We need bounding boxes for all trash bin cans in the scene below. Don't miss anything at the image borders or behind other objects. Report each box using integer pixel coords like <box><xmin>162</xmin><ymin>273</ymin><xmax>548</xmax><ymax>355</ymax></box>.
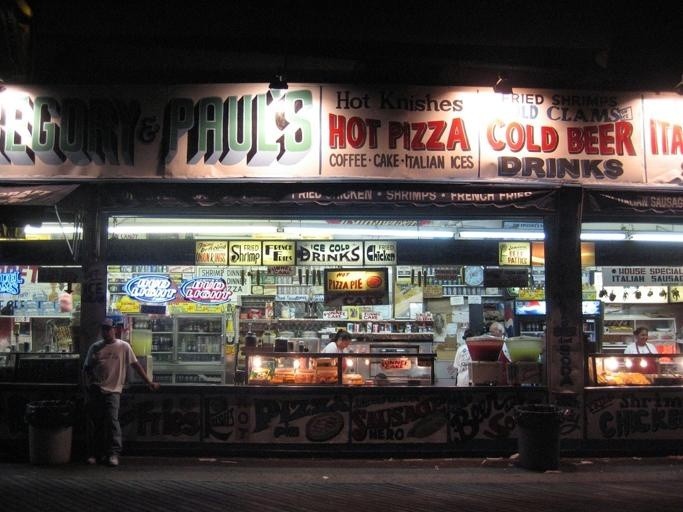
<box><xmin>27</xmin><ymin>400</ymin><xmax>73</xmax><ymax>465</ymax></box>
<box><xmin>513</xmin><ymin>404</ymin><xmax>564</xmax><ymax>472</ymax></box>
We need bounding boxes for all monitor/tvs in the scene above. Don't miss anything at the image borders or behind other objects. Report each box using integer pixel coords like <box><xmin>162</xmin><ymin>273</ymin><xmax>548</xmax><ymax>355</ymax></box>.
<box><xmin>323</xmin><ymin>267</ymin><xmax>390</xmax><ymax>306</ymax></box>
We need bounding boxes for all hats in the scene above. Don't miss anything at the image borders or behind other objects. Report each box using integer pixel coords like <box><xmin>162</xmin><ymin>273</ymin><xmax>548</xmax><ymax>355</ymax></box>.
<box><xmin>101</xmin><ymin>318</ymin><xmax>116</xmax><ymax>328</ymax></box>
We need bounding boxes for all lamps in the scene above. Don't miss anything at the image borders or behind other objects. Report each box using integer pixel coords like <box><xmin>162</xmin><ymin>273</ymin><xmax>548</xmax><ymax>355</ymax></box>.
<box><xmin>493</xmin><ymin>78</ymin><xmax>512</xmax><ymax>97</ymax></box>
<box><xmin>269</xmin><ymin>75</ymin><xmax>289</xmax><ymax>101</ymax></box>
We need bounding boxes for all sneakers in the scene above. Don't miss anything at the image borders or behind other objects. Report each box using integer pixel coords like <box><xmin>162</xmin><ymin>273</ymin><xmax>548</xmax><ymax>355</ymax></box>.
<box><xmin>107</xmin><ymin>455</ymin><xmax>118</xmax><ymax>466</ymax></box>
<box><xmin>86</xmin><ymin>457</ymin><xmax>96</xmax><ymax>465</ymax></box>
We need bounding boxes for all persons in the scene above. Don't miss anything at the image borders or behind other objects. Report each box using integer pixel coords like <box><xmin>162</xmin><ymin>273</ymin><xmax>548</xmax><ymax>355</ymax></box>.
<box><xmin>453</xmin><ymin>328</ymin><xmax>477</xmax><ymax>387</ymax></box>
<box><xmin>80</xmin><ymin>317</ymin><xmax>162</xmax><ymax>468</ymax></box>
<box><xmin>623</xmin><ymin>325</ymin><xmax>661</xmax><ymax>376</ymax></box>
<box><xmin>488</xmin><ymin>321</ymin><xmax>513</xmax><ymax>387</ymax></box>
<box><xmin>317</xmin><ymin>328</ymin><xmax>353</xmax><ymax>374</ymax></box>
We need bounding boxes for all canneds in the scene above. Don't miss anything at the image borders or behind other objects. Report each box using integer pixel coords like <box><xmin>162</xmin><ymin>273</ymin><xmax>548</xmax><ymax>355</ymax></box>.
<box><xmin>429</xmin><ymin>278</ymin><xmax>458</xmax><ymax>286</ymax></box>
<box><xmin>344</xmin><ymin>323</ymin><xmax>433</xmax><ymax>333</ymax></box>
<box><xmin>443</xmin><ymin>287</ymin><xmax>481</xmax><ymax>295</ymax></box>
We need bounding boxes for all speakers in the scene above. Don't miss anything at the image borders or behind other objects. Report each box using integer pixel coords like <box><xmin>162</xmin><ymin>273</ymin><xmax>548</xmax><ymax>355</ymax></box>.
<box><xmin>38</xmin><ymin>267</ymin><xmax>83</xmax><ymax>282</ymax></box>
<box><xmin>483</xmin><ymin>269</ymin><xmax>528</xmax><ymax>287</ymax></box>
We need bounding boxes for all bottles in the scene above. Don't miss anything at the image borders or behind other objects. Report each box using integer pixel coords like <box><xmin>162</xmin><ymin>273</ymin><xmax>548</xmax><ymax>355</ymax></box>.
<box><xmin>422</xmin><ymin>267</ymin><xmax>481</xmax><ymax>295</ymax></box>
<box><xmin>133</xmin><ymin>316</ymin><xmax>221</xmax><ymax>384</ymax></box>
<box><xmin>520</xmin><ymin>318</ymin><xmax>545</xmax><ymax>332</ymax></box>
<box><xmin>518</xmin><ymin>286</ymin><xmax>544</xmax><ymax>299</ymax></box>
<box><xmin>581</xmin><ymin>283</ymin><xmax>597</xmax><ymax>301</ymax></box>
